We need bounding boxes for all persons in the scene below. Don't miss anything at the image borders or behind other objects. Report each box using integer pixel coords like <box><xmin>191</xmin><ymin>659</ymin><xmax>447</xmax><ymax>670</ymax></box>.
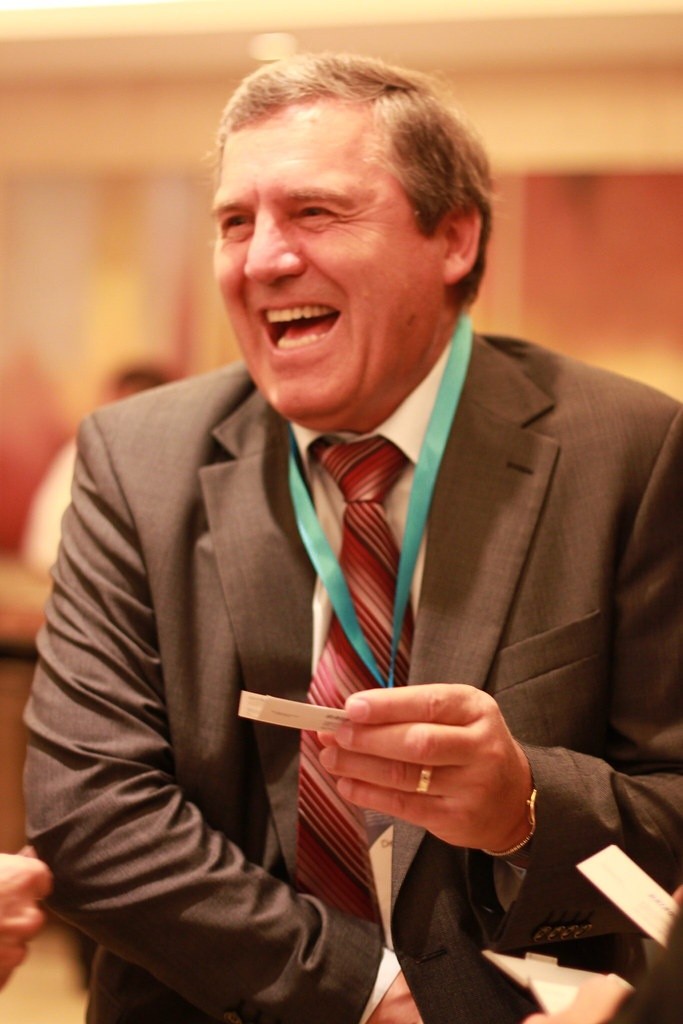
<box><xmin>24</xmin><ymin>367</ymin><xmax>165</xmax><ymax>583</ymax></box>
<box><xmin>23</xmin><ymin>56</ymin><xmax>683</xmax><ymax>1024</ymax></box>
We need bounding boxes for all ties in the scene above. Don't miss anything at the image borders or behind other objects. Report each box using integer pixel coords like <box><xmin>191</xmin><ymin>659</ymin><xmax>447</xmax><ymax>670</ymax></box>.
<box><xmin>295</xmin><ymin>432</ymin><xmax>413</xmax><ymax>949</ymax></box>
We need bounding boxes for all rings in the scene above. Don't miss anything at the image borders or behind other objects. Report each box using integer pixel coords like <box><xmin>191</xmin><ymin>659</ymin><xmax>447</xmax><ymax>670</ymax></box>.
<box><xmin>416</xmin><ymin>766</ymin><xmax>432</xmax><ymax>793</ymax></box>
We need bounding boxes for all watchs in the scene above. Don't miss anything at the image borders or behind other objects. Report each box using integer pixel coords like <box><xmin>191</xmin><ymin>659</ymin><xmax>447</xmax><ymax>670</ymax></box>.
<box><xmin>482</xmin><ymin>789</ymin><xmax>537</xmax><ymax>856</ymax></box>
